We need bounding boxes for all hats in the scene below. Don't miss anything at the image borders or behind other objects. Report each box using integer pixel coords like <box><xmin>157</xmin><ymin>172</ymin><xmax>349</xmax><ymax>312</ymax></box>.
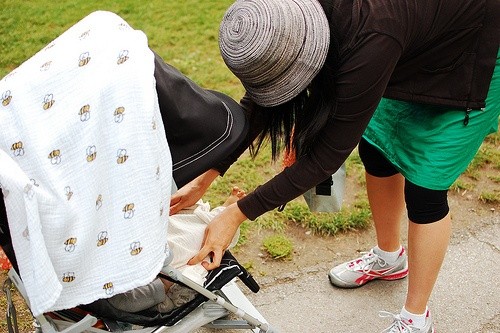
<box><xmin>217</xmin><ymin>0</ymin><xmax>331</xmax><ymax>108</ymax></box>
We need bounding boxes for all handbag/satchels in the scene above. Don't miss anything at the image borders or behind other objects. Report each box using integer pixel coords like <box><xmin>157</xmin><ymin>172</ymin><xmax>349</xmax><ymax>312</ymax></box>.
<box><xmin>302</xmin><ymin>162</ymin><xmax>347</xmax><ymax>214</ymax></box>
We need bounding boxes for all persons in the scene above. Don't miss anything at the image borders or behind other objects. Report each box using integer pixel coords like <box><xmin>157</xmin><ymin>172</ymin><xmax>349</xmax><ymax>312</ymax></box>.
<box><xmin>105</xmin><ymin>186</ymin><xmax>247</xmax><ymax>313</ymax></box>
<box><xmin>167</xmin><ymin>0</ymin><xmax>500</xmax><ymax>333</ymax></box>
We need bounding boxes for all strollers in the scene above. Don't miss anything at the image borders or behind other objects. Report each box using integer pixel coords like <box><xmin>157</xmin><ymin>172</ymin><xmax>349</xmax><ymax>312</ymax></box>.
<box><xmin>1</xmin><ymin>11</ymin><xmax>273</xmax><ymax>333</ymax></box>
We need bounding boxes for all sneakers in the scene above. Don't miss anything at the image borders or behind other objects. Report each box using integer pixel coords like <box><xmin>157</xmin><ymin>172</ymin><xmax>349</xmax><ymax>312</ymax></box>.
<box><xmin>327</xmin><ymin>245</ymin><xmax>408</xmax><ymax>289</ymax></box>
<box><xmin>385</xmin><ymin>309</ymin><xmax>436</xmax><ymax>333</ymax></box>
